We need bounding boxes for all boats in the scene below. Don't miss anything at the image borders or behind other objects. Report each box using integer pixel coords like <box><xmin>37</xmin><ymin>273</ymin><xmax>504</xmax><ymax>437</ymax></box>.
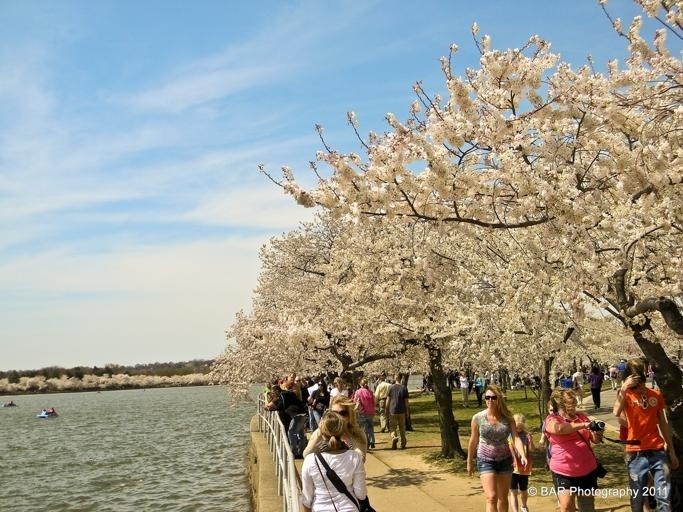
<box><xmin>37</xmin><ymin>407</ymin><xmax>58</xmax><ymax>419</ymax></box>
<box><xmin>1</xmin><ymin>401</ymin><xmax>17</xmax><ymax>408</ymax></box>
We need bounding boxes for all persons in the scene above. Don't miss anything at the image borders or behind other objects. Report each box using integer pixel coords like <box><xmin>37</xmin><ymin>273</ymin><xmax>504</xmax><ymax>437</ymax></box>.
<box><xmin>420</xmin><ymin>359</ymin><xmax>679</xmax><ymax>512</ymax></box>
<box><xmin>41</xmin><ymin>407</ymin><xmax>54</xmax><ymax>415</ymax></box>
<box><xmin>261</xmin><ymin>370</ymin><xmax>411</xmax><ymax>512</ymax></box>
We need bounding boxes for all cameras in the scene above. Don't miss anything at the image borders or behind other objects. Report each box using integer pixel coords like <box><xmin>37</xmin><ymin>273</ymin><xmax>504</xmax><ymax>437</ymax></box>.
<box><xmin>589</xmin><ymin>421</ymin><xmax>605</xmax><ymax>431</ymax></box>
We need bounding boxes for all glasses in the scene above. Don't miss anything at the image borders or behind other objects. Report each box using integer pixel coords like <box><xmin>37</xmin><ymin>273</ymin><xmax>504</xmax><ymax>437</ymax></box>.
<box><xmin>485</xmin><ymin>395</ymin><xmax>497</xmax><ymax>400</ymax></box>
<box><xmin>641</xmin><ymin>394</ymin><xmax>648</xmax><ymax>410</ymax></box>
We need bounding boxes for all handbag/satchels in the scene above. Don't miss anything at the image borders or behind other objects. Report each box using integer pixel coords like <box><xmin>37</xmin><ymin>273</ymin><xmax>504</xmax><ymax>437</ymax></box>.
<box><xmin>597</xmin><ymin>464</ymin><xmax>607</xmax><ymax>478</ymax></box>
